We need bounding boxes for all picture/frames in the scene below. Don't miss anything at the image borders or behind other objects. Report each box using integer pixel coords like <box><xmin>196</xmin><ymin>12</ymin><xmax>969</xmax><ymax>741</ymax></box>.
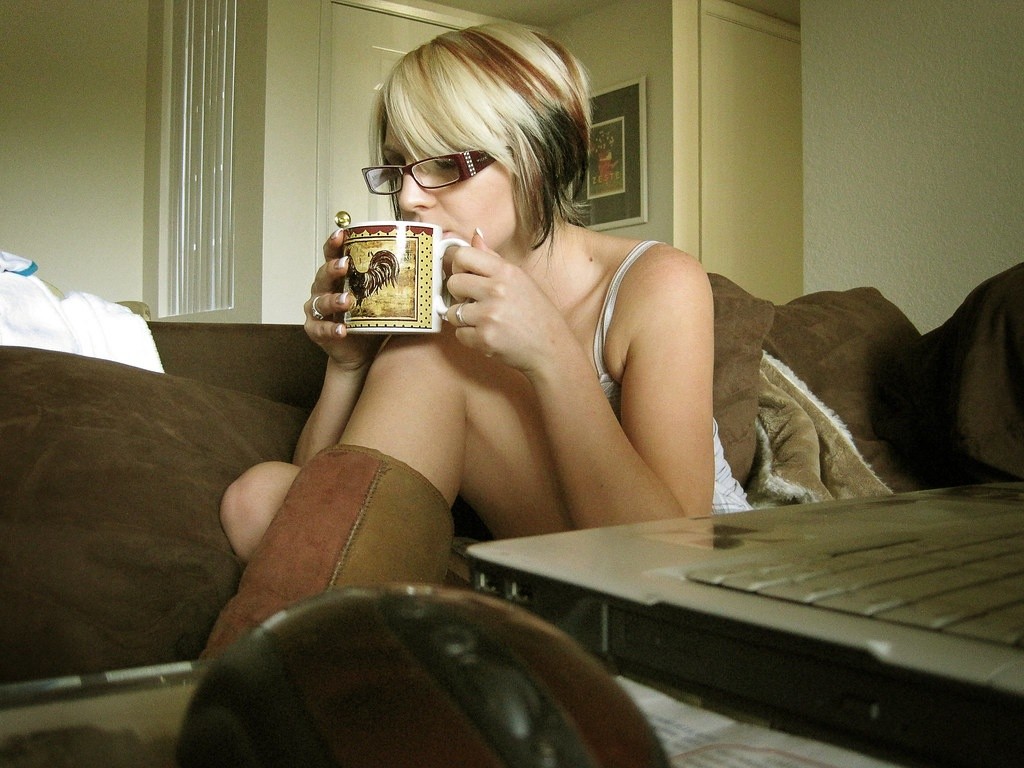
<box><xmin>569</xmin><ymin>76</ymin><xmax>649</xmax><ymax>232</ymax></box>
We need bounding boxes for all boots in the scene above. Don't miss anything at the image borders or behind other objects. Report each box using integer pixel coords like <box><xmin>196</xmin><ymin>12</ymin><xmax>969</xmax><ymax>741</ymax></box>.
<box><xmin>199</xmin><ymin>441</ymin><xmax>457</xmax><ymax>673</ymax></box>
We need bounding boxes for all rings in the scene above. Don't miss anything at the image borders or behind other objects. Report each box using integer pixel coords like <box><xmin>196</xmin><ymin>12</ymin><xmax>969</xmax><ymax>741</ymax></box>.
<box><xmin>455</xmin><ymin>302</ymin><xmax>468</xmax><ymax>327</ymax></box>
<box><xmin>312</xmin><ymin>296</ymin><xmax>326</xmax><ymax>321</ymax></box>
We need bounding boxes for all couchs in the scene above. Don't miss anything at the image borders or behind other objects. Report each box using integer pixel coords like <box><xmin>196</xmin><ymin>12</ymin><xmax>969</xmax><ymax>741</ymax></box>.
<box><xmin>0</xmin><ymin>261</ymin><xmax>1023</xmax><ymax>686</ymax></box>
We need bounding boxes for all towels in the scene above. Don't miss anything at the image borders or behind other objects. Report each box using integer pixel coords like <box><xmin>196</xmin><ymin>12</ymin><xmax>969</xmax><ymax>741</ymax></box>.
<box><xmin>2</xmin><ymin>249</ymin><xmax>165</xmax><ymax>371</ymax></box>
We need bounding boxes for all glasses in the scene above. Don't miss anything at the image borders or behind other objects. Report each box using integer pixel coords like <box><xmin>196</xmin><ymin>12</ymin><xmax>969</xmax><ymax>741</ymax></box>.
<box><xmin>362</xmin><ymin>151</ymin><xmax>496</xmax><ymax>195</ymax></box>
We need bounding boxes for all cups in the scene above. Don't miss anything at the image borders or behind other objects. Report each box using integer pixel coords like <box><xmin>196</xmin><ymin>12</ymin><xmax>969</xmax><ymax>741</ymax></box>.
<box><xmin>341</xmin><ymin>220</ymin><xmax>472</xmax><ymax>335</ymax></box>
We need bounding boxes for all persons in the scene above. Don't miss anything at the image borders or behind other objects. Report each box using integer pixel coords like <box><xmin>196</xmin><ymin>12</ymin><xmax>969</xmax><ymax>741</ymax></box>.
<box><xmin>201</xmin><ymin>20</ymin><xmax>752</xmax><ymax>668</ymax></box>
